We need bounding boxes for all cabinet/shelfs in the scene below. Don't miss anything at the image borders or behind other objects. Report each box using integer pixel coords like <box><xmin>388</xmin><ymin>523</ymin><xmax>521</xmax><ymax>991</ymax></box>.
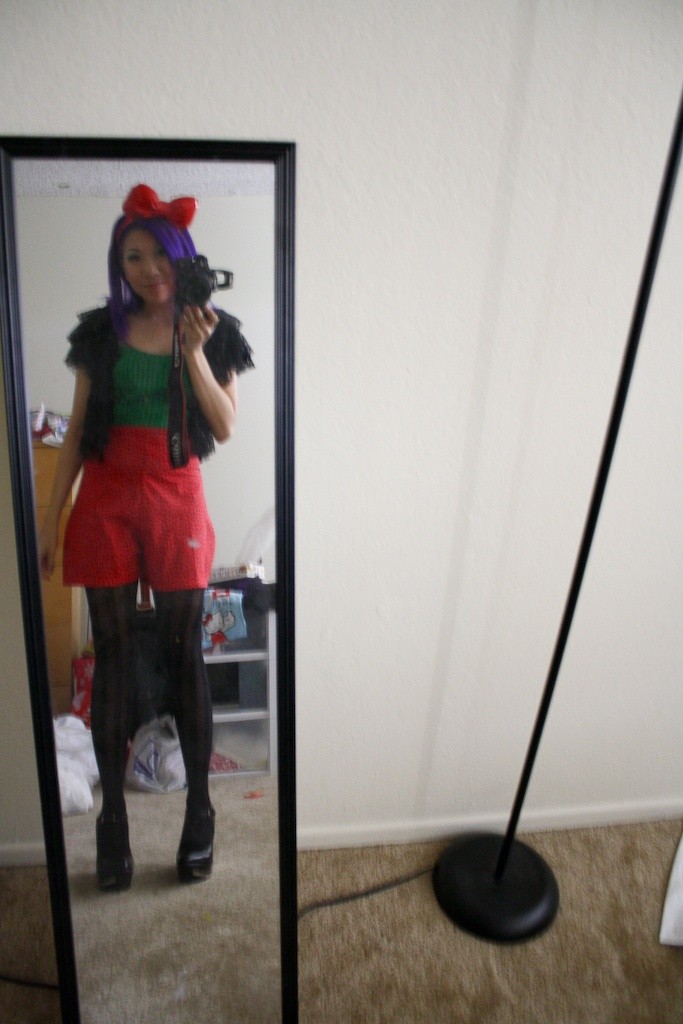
<box><xmin>204</xmin><ymin>610</ymin><xmax>278</xmax><ymax>777</ymax></box>
<box><xmin>33</xmin><ymin>440</ymin><xmax>87</xmax><ymax>718</ymax></box>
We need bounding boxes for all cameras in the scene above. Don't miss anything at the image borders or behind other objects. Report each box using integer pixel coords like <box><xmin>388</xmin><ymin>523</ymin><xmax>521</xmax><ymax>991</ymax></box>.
<box><xmin>176</xmin><ymin>255</ymin><xmax>234</xmax><ymax>307</ymax></box>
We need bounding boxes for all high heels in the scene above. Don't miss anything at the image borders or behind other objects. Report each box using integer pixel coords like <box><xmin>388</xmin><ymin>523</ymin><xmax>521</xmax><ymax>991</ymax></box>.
<box><xmin>174</xmin><ymin>809</ymin><xmax>216</xmax><ymax>886</ymax></box>
<box><xmin>93</xmin><ymin>813</ymin><xmax>134</xmax><ymax>892</ymax></box>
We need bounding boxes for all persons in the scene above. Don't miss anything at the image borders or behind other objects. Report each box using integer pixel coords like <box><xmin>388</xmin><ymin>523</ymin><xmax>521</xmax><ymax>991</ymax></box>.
<box><xmin>35</xmin><ymin>186</ymin><xmax>258</xmax><ymax>887</ymax></box>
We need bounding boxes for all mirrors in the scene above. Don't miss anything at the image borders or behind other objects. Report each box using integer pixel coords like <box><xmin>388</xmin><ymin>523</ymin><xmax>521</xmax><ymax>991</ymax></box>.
<box><xmin>0</xmin><ymin>133</ymin><xmax>300</xmax><ymax>1024</ymax></box>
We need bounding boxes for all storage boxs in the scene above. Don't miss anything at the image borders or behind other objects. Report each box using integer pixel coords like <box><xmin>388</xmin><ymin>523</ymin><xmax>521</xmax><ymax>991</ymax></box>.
<box><xmin>210</xmin><ymin>563</ymin><xmax>265</xmax><ymax>583</ymax></box>
